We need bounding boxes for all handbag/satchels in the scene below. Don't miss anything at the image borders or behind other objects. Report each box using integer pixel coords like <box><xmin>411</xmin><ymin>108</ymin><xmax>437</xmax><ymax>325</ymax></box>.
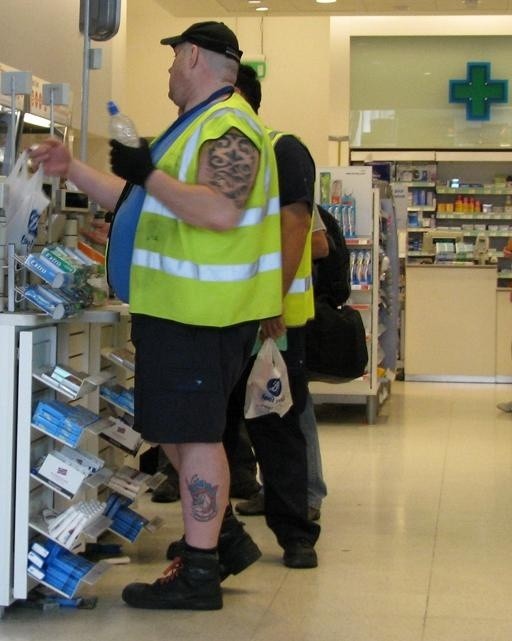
<box><xmin>299</xmin><ymin>302</ymin><xmax>370</xmax><ymax>387</ymax></box>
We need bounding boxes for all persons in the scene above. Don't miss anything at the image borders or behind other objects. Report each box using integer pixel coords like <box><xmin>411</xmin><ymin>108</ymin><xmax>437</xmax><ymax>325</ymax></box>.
<box><xmin>234</xmin><ymin>205</ymin><xmax>332</xmax><ymax>515</ymax></box>
<box><xmin>139</xmin><ymin>425</ymin><xmax>259</xmax><ymax>505</ymax></box>
<box><xmin>167</xmin><ymin>65</ymin><xmax>326</xmax><ymax>569</ymax></box>
<box><xmin>27</xmin><ymin>21</ymin><xmax>285</xmax><ymax>609</ymax></box>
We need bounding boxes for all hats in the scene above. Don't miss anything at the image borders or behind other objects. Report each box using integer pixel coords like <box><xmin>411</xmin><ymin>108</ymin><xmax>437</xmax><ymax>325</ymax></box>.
<box><xmin>159</xmin><ymin>20</ymin><xmax>243</xmax><ymax>67</ymax></box>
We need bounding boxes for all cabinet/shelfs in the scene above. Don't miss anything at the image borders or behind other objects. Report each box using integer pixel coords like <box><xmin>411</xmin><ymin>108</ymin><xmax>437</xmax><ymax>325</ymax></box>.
<box><xmin>350</xmin><ymin>152</ymin><xmax>512</xmax><ymax>266</ymax></box>
<box><xmin>310</xmin><ymin>167</ymin><xmax>398</xmax><ymax>424</ymax></box>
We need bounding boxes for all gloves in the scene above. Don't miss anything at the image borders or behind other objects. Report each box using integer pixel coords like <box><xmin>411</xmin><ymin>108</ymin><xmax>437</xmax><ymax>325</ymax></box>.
<box><xmin>108</xmin><ymin>137</ymin><xmax>157</xmax><ymax>188</ymax></box>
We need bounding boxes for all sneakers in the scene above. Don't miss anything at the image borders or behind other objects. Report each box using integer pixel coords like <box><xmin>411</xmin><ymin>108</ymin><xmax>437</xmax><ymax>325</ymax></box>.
<box><xmin>234</xmin><ymin>493</ymin><xmax>267</xmax><ymax>516</ymax></box>
<box><xmin>217</xmin><ymin>520</ymin><xmax>264</xmax><ymax>586</ymax></box>
<box><xmin>151</xmin><ymin>472</ymin><xmax>181</xmax><ymax>503</ymax></box>
<box><xmin>165</xmin><ymin>533</ymin><xmax>187</xmax><ymax>561</ymax></box>
<box><xmin>119</xmin><ymin>562</ymin><xmax>224</xmax><ymax>612</ymax></box>
<box><xmin>228</xmin><ymin>477</ymin><xmax>263</xmax><ymax>500</ymax></box>
<box><xmin>306</xmin><ymin>504</ymin><xmax>321</xmax><ymax>522</ymax></box>
<box><xmin>282</xmin><ymin>535</ymin><xmax>320</xmax><ymax>569</ymax></box>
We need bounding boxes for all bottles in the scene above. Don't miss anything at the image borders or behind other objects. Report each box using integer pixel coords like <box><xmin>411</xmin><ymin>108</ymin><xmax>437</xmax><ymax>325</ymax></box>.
<box><xmin>106</xmin><ymin>100</ymin><xmax>140</xmax><ymax>148</ymax></box>
<box><xmin>454</xmin><ymin>193</ymin><xmax>482</xmax><ymax>213</ymax></box>
<box><xmin>504</xmin><ymin>195</ymin><xmax>512</xmax><ymax>213</ymax></box>
